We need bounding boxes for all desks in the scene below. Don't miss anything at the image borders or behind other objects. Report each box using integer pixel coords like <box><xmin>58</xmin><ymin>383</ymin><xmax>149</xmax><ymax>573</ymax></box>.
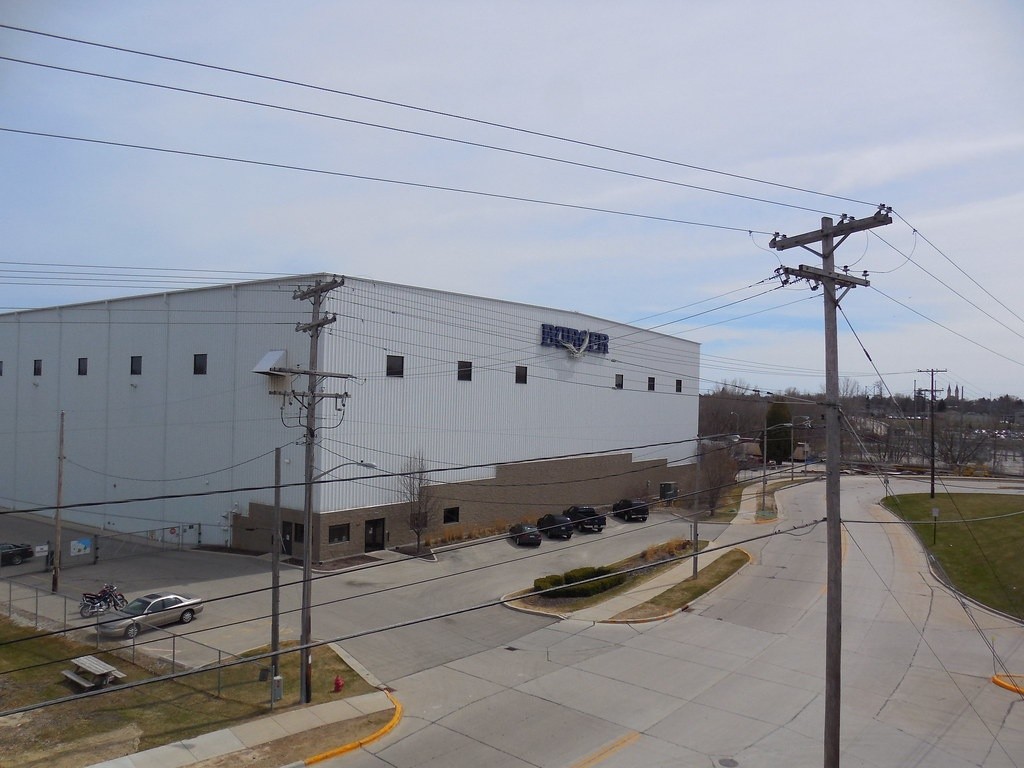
<box><xmin>70</xmin><ymin>655</ymin><xmax>116</xmax><ymax>691</ymax></box>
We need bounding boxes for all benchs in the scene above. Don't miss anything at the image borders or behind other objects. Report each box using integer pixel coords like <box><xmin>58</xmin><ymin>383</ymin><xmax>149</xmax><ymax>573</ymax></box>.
<box><xmin>111</xmin><ymin>669</ymin><xmax>128</xmax><ymax>680</ymax></box>
<box><xmin>63</xmin><ymin>670</ymin><xmax>96</xmax><ymax>694</ymax></box>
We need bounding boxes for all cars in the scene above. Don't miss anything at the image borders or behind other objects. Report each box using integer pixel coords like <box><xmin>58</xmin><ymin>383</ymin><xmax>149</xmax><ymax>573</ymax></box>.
<box><xmin>95</xmin><ymin>589</ymin><xmax>204</xmax><ymax>641</ymax></box>
<box><xmin>510</xmin><ymin>523</ymin><xmax>542</xmax><ymax>547</ymax></box>
<box><xmin>0</xmin><ymin>543</ymin><xmax>35</xmax><ymax>567</ymax></box>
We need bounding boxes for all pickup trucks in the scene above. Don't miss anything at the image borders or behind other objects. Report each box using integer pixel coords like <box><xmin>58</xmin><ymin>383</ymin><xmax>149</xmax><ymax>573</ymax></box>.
<box><xmin>563</xmin><ymin>505</ymin><xmax>606</xmax><ymax>532</ymax></box>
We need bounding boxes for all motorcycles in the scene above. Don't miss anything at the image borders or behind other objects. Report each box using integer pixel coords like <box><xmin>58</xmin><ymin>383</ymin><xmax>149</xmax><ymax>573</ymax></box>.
<box><xmin>80</xmin><ymin>584</ymin><xmax>128</xmax><ymax>618</ymax></box>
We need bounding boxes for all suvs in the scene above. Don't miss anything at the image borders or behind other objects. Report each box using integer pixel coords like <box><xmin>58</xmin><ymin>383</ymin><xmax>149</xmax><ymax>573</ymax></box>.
<box><xmin>537</xmin><ymin>513</ymin><xmax>574</xmax><ymax>539</ymax></box>
<box><xmin>613</xmin><ymin>496</ymin><xmax>649</xmax><ymax>522</ymax></box>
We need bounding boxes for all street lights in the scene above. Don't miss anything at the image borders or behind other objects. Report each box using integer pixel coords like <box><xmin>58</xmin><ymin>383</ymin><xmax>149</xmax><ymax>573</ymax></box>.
<box><xmin>731</xmin><ymin>411</ymin><xmax>740</xmax><ymax>432</ymax></box>
<box><xmin>761</xmin><ymin>422</ymin><xmax>794</xmax><ymax>512</ymax></box>
<box><xmin>304</xmin><ymin>459</ymin><xmax>378</xmax><ymax>703</ymax></box>
<box><xmin>791</xmin><ymin>415</ymin><xmax>810</xmax><ymax>480</ymax></box>
<box><xmin>693</xmin><ymin>431</ymin><xmax>742</xmax><ymax>580</ymax></box>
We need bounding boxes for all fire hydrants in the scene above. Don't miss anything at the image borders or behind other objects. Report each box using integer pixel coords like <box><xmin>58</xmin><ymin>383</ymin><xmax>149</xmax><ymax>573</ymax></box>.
<box><xmin>334</xmin><ymin>674</ymin><xmax>345</xmax><ymax>693</ymax></box>
